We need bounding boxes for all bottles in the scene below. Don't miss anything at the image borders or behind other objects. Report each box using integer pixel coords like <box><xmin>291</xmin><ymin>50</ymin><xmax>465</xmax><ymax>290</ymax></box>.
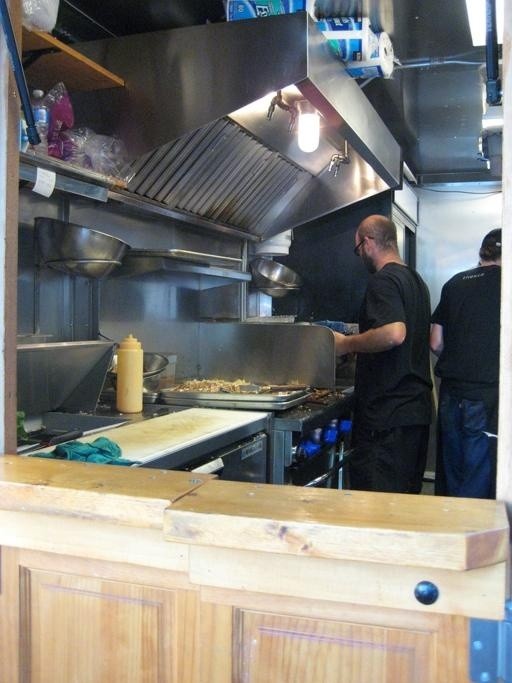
<box><xmin>116</xmin><ymin>336</ymin><xmax>143</xmax><ymax>415</ymax></box>
<box><xmin>28</xmin><ymin>88</ymin><xmax>50</xmax><ymax>159</ymax></box>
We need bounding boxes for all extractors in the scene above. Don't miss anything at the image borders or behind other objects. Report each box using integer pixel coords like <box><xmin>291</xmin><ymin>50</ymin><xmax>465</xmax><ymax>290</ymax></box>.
<box><xmin>79</xmin><ymin>89</ymin><xmax>403</xmax><ymax>243</ymax></box>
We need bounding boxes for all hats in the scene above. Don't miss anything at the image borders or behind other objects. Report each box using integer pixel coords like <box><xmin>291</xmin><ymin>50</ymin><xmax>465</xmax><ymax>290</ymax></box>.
<box><xmin>484</xmin><ymin>229</ymin><xmax>501</xmax><ymax>248</ymax></box>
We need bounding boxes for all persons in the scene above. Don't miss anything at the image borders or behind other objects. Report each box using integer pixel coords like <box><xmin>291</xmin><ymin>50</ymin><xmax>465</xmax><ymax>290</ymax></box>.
<box><xmin>429</xmin><ymin>227</ymin><xmax>500</xmax><ymax>498</ymax></box>
<box><xmin>326</xmin><ymin>214</ymin><xmax>433</xmax><ymax>491</ymax></box>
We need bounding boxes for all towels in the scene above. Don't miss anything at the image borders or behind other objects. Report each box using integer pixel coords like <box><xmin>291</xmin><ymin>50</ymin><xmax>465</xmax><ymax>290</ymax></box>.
<box><xmin>28</xmin><ymin>437</ymin><xmax>143</xmax><ymax>466</ymax></box>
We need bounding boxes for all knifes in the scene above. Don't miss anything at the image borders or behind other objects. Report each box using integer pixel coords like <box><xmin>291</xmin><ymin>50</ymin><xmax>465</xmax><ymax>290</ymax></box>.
<box><xmin>16</xmin><ymin>426</ymin><xmax>82</xmax><ymax>455</ymax></box>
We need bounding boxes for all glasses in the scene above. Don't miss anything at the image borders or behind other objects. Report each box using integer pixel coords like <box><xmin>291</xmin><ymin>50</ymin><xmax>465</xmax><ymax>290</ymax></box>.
<box><xmin>354</xmin><ymin>236</ymin><xmax>372</xmax><ymax>257</ymax></box>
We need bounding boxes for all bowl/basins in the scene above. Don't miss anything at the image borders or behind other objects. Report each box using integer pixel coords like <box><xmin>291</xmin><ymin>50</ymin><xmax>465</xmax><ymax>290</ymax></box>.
<box><xmin>250</xmin><ymin>257</ymin><xmax>303</xmax><ymax>300</ymax></box>
<box><xmin>335</xmin><ymin>331</ymin><xmax>358</xmax><ymax>370</ymax></box>
<box><xmin>34</xmin><ymin>216</ymin><xmax>132</xmax><ymax>281</ymax></box>
<box><xmin>107</xmin><ymin>351</ymin><xmax>169</xmax><ymax>392</ymax></box>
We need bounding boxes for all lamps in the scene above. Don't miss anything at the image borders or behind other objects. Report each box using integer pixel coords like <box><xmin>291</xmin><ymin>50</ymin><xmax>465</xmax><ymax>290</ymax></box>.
<box><xmin>294</xmin><ymin>99</ymin><xmax>323</xmax><ymax>153</ymax></box>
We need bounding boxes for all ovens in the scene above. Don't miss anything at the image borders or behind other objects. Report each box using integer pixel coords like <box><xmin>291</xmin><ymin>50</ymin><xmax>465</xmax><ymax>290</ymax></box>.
<box><xmin>286</xmin><ymin>415</ymin><xmax>352</xmax><ymax>491</ymax></box>
<box><xmin>169</xmin><ymin>432</ymin><xmax>268</xmax><ymax>486</ymax></box>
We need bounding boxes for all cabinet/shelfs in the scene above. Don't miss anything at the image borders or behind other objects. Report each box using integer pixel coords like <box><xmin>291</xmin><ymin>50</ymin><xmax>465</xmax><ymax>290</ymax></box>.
<box><xmin>20</xmin><ymin>19</ymin><xmax>128</xmax><ymax>191</ymax></box>
